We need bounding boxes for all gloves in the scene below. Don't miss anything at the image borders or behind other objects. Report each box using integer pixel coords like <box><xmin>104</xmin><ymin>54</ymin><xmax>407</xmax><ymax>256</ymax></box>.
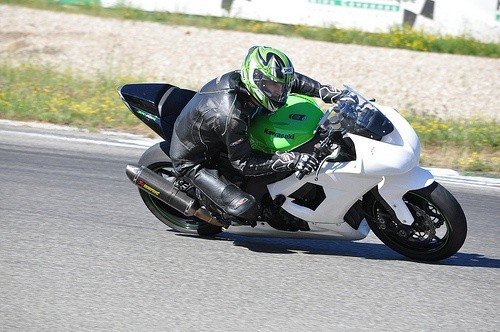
<box><xmin>319</xmin><ymin>84</ymin><xmax>358</xmax><ymax>104</ymax></box>
<box><xmin>271</xmin><ymin>151</ymin><xmax>320</xmax><ymax>176</ymax></box>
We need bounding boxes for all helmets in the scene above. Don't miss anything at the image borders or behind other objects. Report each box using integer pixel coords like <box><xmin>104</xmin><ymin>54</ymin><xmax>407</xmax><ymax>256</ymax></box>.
<box><xmin>241</xmin><ymin>45</ymin><xmax>293</xmax><ymax>112</ymax></box>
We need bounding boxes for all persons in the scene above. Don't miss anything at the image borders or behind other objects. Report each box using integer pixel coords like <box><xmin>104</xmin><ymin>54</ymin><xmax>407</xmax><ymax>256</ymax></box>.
<box><xmin>169</xmin><ymin>43</ymin><xmax>360</xmax><ymax>231</ymax></box>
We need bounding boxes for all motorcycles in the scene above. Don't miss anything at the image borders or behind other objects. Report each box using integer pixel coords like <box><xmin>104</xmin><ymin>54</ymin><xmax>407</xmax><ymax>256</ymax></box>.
<box><xmin>115</xmin><ymin>83</ymin><xmax>468</xmax><ymax>264</ymax></box>
